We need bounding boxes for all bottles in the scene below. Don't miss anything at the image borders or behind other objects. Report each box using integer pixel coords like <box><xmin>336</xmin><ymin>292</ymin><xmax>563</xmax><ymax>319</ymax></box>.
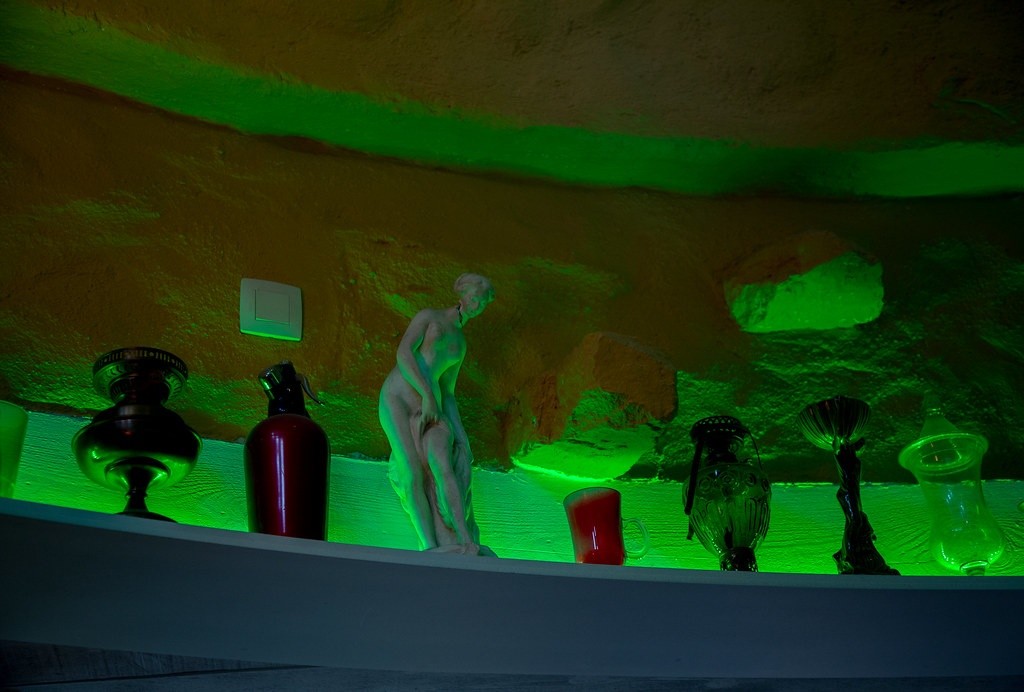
<box><xmin>896</xmin><ymin>380</ymin><xmax>1006</xmax><ymax>576</ymax></box>
<box><xmin>240</xmin><ymin>357</ymin><xmax>330</xmax><ymax>543</ymax></box>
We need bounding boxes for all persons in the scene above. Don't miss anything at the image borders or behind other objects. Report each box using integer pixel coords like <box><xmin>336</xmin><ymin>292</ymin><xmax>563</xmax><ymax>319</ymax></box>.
<box><xmin>378</xmin><ymin>272</ymin><xmax>494</xmax><ymax>552</ymax></box>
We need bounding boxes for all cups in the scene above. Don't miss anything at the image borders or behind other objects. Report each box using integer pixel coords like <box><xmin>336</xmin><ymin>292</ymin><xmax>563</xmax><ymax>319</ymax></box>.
<box><xmin>560</xmin><ymin>487</ymin><xmax>628</xmax><ymax>565</ymax></box>
